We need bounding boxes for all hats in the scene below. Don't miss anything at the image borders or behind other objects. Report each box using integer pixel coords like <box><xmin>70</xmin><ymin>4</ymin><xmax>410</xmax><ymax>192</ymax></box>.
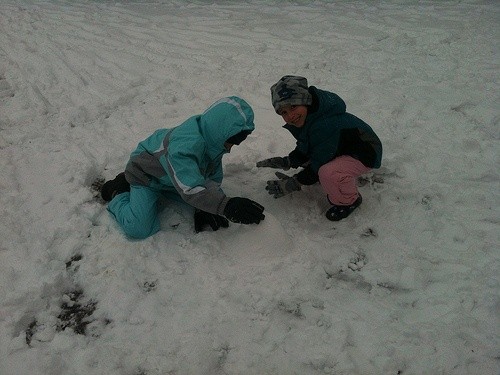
<box><xmin>271</xmin><ymin>75</ymin><xmax>314</xmax><ymax>114</ymax></box>
<box><xmin>226</xmin><ymin>129</ymin><xmax>253</xmax><ymax>146</ymax></box>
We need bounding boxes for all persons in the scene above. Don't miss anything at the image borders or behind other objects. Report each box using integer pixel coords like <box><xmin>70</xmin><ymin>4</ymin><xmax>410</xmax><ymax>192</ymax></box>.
<box><xmin>101</xmin><ymin>96</ymin><xmax>265</xmax><ymax>240</ymax></box>
<box><xmin>256</xmin><ymin>75</ymin><xmax>383</xmax><ymax>221</ymax></box>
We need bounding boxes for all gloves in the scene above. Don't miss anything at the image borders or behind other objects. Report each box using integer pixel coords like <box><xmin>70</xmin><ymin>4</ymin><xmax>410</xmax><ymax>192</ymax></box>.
<box><xmin>194</xmin><ymin>208</ymin><xmax>229</xmax><ymax>233</ymax></box>
<box><xmin>266</xmin><ymin>171</ymin><xmax>303</xmax><ymax>199</ymax></box>
<box><xmin>223</xmin><ymin>196</ymin><xmax>265</xmax><ymax>225</ymax></box>
<box><xmin>256</xmin><ymin>156</ymin><xmax>291</xmax><ymax>171</ymax></box>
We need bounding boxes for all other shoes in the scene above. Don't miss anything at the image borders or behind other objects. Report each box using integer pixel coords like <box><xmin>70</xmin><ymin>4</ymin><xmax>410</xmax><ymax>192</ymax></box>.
<box><xmin>326</xmin><ymin>192</ymin><xmax>362</xmax><ymax>221</ymax></box>
<box><xmin>101</xmin><ymin>172</ymin><xmax>130</xmax><ymax>201</ymax></box>
